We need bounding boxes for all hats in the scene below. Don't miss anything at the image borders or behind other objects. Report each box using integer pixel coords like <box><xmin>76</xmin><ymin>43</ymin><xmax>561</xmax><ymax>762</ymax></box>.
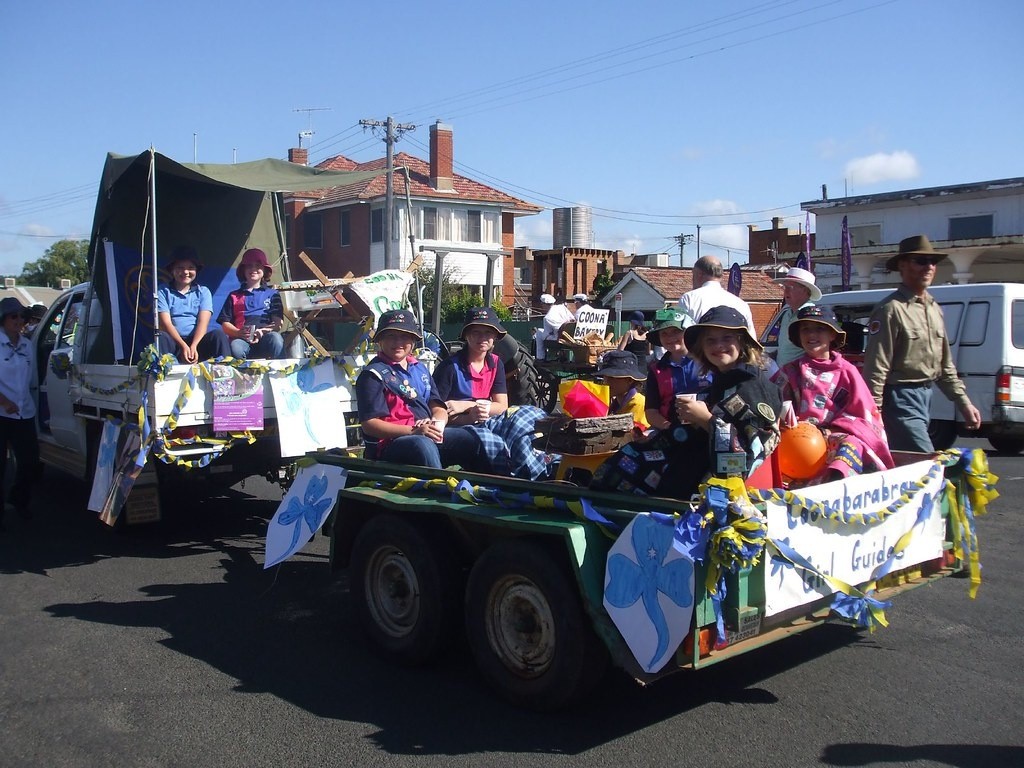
<box><xmin>684</xmin><ymin>305</ymin><xmax>764</xmax><ymax>360</ymax></box>
<box><xmin>540</xmin><ymin>294</ymin><xmax>556</xmax><ymax>304</ymax></box>
<box><xmin>592</xmin><ymin>351</ymin><xmax>648</xmax><ymax>381</ymax></box>
<box><xmin>629</xmin><ymin>310</ymin><xmax>644</xmax><ymax>326</ymax></box>
<box><xmin>788</xmin><ymin>306</ymin><xmax>846</xmax><ymax>350</ymax></box>
<box><xmin>773</xmin><ymin>268</ymin><xmax>823</xmax><ymax>301</ymax></box>
<box><xmin>29</xmin><ymin>304</ymin><xmax>48</xmax><ymax>320</ymax></box>
<box><xmin>459</xmin><ymin>305</ymin><xmax>508</xmax><ymax>341</ymax></box>
<box><xmin>236</xmin><ymin>248</ymin><xmax>272</xmax><ymax>284</ymax></box>
<box><xmin>0</xmin><ymin>297</ymin><xmax>32</xmax><ymax>327</ymax></box>
<box><xmin>166</xmin><ymin>246</ymin><xmax>204</xmax><ymax>276</ymax></box>
<box><xmin>646</xmin><ymin>307</ymin><xmax>692</xmax><ymax>347</ymax></box>
<box><xmin>371</xmin><ymin>310</ymin><xmax>423</xmax><ymax>342</ymax></box>
<box><xmin>887</xmin><ymin>233</ymin><xmax>949</xmax><ymax>272</ymax></box>
<box><xmin>573</xmin><ymin>294</ymin><xmax>588</xmax><ymax>303</ymax></box>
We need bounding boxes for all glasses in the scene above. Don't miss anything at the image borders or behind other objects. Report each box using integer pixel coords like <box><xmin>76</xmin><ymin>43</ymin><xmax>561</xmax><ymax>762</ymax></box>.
<box><xmin>902</xmin><ymin>256</ymin><xmax>936</xmax><ymax>266</ymax></box>
<box><xmin>780</xmin><ymin>285</ymin><xmax>803</xmax><ymax>291</ymax></box>
<box><xmin>4</xmin><ymin>313</ymin><xmax>26</xmax><ymax>320</ymax></box>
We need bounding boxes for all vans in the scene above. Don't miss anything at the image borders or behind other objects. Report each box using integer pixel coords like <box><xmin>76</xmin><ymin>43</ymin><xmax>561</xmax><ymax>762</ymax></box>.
<box><xmin>759</xmin><ymin>281</ymin><xmax>1024</xmax><ymax>453</ymax></box>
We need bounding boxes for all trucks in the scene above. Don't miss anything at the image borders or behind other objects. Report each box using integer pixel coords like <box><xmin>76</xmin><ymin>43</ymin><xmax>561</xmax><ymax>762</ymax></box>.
<box><xmin>26</xmin><ymin>281</ymin><xmax>436</xmax><ymax>521</ymax></box>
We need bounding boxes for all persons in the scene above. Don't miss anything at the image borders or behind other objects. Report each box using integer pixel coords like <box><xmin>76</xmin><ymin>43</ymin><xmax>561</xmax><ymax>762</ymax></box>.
<box><xmin>767</xmin><ymin>305</ymin><xmax>894</xmax><ymax>481</ymax></box>
<box><xmin>157</xmin><ymin>247</ymin><xmax>231</xmax><ymax>364</ymax></box>
<box><xmin>431</xmin><ymin>308</ymin><xmax>556</xmax><ymax>478</ymax></box>
<box><xmin>862</xmin><ymin>234</ymin><xmax>981</xmax><ymax>453</ymax></box>
<box><xmin>535</xmin><ymin>294</ymin><xmax>574</xmax><ymax>359</ymax></box>
<box><xmin>618</xmin><ymin>311</ymin><xmax>649</xmax><ymax>350</ymax></box>
<box><xmin>0</xmin><ymin>298</ymin><xmax>56</xmax><ymax>517</ymax></box>
<box><xmin>677</xmin><ymin>256</ymin><xmax>756</xmax><ymax>341</ymax></box>
<box><xmin>591</xmin><ymin>350</ymin><xmax>652</xmax><ymax>429</ymax></box>
<box><xmin>572</xmin><ymin>294</ymin><xmax>594</xmax><ymax>318</ymax></box>
<box><xmin>761</xmin><ymin>268</ymin><xmax>821</xmax><ymax>367</ymax></box>
<box><xmin>598</xmin><ymin>304</ymin><xmax>780</xmax><ymax>494</ymax></box>
<box><xmin>646</xmin><ymin>306</ymin><xmax>712</xmax><ymax>429</ymax></box>
<box><xmin>216</xmin><ymin>249</ymin><xmax>284</xmax><ymax>359</ymax></box>
<box><xmin>355</xmin><ymin>310</ymin><xmax>483</xmax><ymax>469</ymax></box>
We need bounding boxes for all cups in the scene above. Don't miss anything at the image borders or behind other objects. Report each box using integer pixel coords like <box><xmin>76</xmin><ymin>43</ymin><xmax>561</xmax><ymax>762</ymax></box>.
<box><xmin>242</xmin><ymin>324</ymin><xmax>255</xmax><ymax>342</ymax></box>
<box><xmin>675</xmin><ymin>394</ymin><xmax>696</xmax><ymax>425</ymax></box>
<box><xmin>428</xmin><ymin>420</ymin><xmax>445</xmax><ymax>444</ymax></box>
<box><xmin>476</xmin><ymin>399</ymin><xmax>492</xmax><ymax>422</ymax></box>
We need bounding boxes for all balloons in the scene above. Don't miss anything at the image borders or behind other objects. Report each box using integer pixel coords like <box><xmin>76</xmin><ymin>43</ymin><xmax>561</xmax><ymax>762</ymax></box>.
<box><xmin>778</xmin><ymin>424</ymin><xmax>827</xmax><ymax>480</ymax></box>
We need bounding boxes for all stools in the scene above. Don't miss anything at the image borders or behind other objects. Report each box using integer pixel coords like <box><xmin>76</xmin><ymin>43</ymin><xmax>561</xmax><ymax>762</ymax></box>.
<box><xmin>554</xmin><ymin>455</ymin><xmax>617</xmax><ymax>485</ymax></box>
